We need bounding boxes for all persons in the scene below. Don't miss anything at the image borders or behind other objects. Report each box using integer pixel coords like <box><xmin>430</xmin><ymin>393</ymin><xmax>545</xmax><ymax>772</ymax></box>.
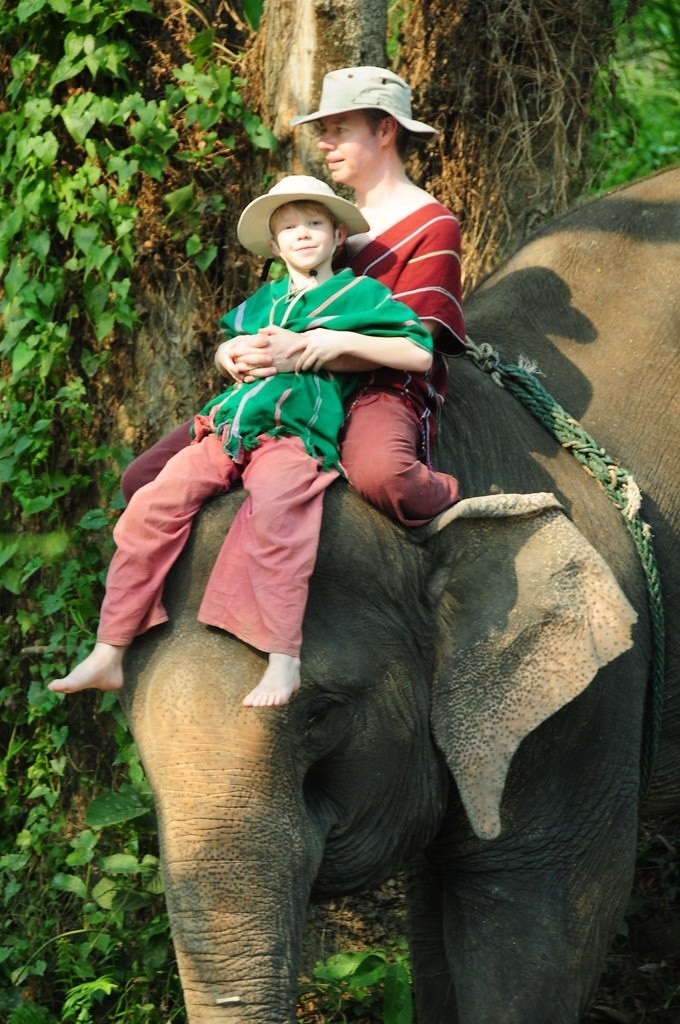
<box><xmin>120</xmin><ymin>66</ymin><xmax>466</xmax><ymax>528</ymax></box>
<box><xmin>46</xmin><ymin>175</ymin><xmax>434</xmax><ymax>708</ymax></box>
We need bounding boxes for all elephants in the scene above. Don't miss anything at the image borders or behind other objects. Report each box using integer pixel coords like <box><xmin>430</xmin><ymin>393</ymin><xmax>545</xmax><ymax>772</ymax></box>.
<box><xmin>119</xmin><ymin>161</ymin><xmax>680</xmax><ymax>1024</ymax></box>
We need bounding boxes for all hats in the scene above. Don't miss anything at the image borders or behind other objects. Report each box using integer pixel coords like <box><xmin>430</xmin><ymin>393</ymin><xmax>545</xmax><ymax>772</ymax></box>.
<box><xmin>238</xmin><ymin>175</ymin><xmax>370</xmax><ymax>258</ymax></box>
<box><xmin>291</xmin><ymin>66</ymin><xmax>440</xmax><ymax>144</ymax></box>
